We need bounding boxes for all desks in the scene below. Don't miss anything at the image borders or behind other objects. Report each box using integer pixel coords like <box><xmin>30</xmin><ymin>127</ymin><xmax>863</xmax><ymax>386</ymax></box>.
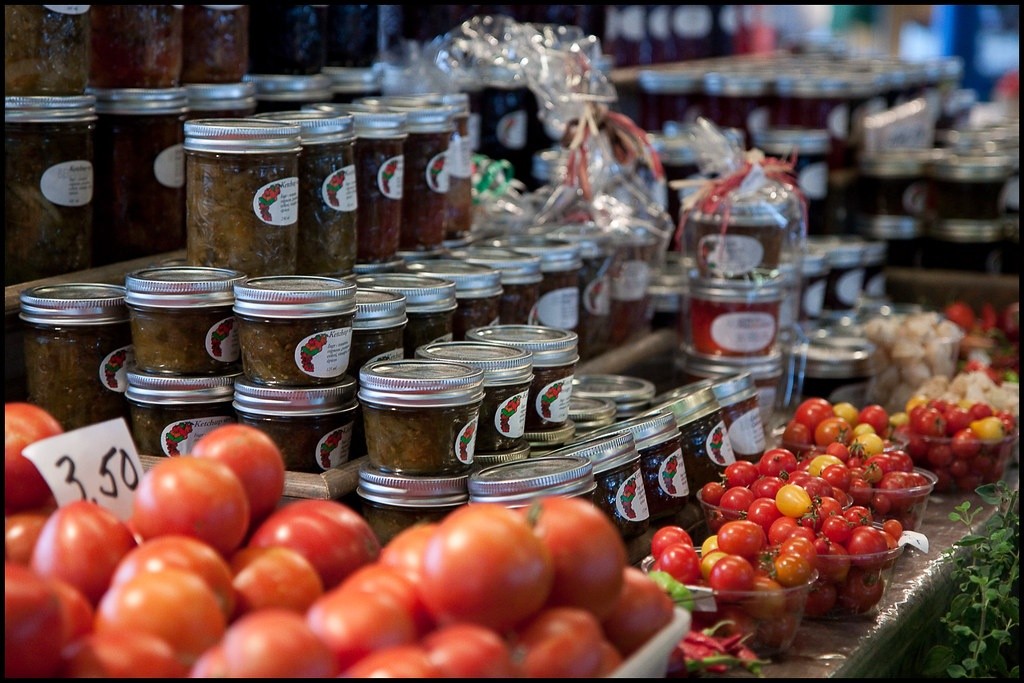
<box><xmin>721</xmin><ymin>468</ymin><xmax>1021</xmax><ymax>680</ymax></box>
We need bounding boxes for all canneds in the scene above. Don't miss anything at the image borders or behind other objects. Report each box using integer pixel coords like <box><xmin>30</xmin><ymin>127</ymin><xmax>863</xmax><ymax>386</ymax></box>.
<box><xmin>1</xmin><ymin>0</ymin><xmax>1022</xmax><ymax>559</ymax></box>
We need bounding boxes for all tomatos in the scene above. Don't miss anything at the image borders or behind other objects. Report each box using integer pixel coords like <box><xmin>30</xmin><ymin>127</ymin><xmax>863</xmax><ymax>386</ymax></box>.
<box><xmin>3</xmin><ymin>395</ymin><xmax>1016</xmax><ymax>677</ymax></box>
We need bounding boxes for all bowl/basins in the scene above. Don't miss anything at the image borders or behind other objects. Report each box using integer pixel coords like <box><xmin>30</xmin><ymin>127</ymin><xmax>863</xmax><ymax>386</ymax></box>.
<box><xmin>696</xmin><ymin>487</ymin><xmax>853</xmax><ymax>537</ymax></box>
<box><xmin>771</xmin><ymin>427</ymin><xmax>910</xmax><ymax>460</ymax></box>
<box><xmin>849</xmin><ymin>467</ymin><xmax>939</xmax><ymax>532</ymax></box>
<box><xmin>641</xmin><ymin>547</ymin><xmax>819</xmax><ymax>657</ymax></box>
<box><xmin>862</xmin><ymin>320</ymin><xmax>967</xmax><ymax>413</ymax></box>
<box><xmin>804</xmin><ymin>521</ymin><xmax>907</xmax><ymax>622</ymax></box>
<box><xmin>901</xmin><ymin>431</ymin><xmax>1018</xmax><ymax>495</ymax></box>
